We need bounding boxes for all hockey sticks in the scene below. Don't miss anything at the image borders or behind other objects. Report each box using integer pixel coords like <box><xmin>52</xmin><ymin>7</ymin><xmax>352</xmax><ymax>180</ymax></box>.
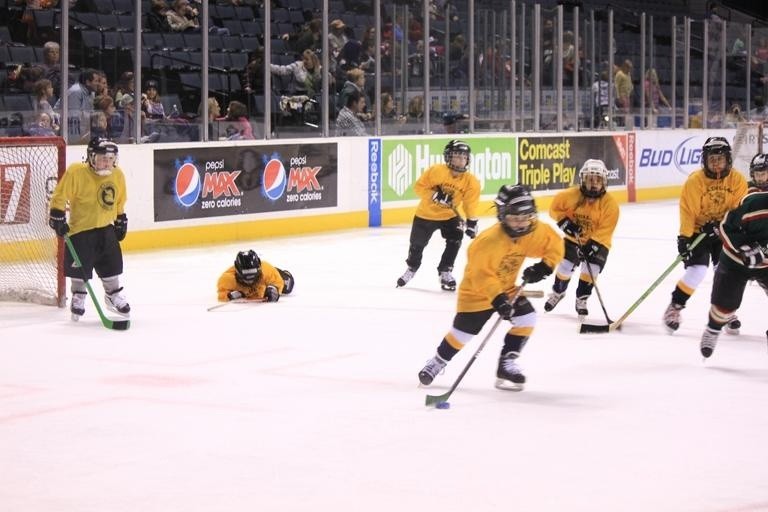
<box><xmin>427</xmin><ymin>279</ymin><xmax>527</xmax><ymax>406</ymax></box>
<box><xmin>207</xmin><ymin>295</ymin><xmax>267</xmax><ymax>313</ymax></box>
<box><xmin>437</xmin><ymin>187</ymin><xmax>481</xmax><ymax>241</ymax></box>
<box><xmin>578</xmin><ymin>237</ymin><xmax>621</xmax><ymax>330</ymax></box>
<box><xmin>65</xmin><ymin>233</ymin><xmax>131</xmax><ymax>330</ymax></box>
<box><xmin>580</xmin><ymin>231</ymin><xmax>709</xmax><ymax>332</ymax></box>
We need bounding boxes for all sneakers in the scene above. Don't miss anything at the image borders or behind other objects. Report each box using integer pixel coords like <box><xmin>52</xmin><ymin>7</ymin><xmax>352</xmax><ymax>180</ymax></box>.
<box><xmin>576</xmin><ymin>297</ymin><xmax>588</xmax><ymax>315</ymax></box>
<box><xmin>397</xmin><ymin>270</ymin><xmax>414</xmax><ymax>286</ymax></box>
<box><xmin>419</xmin><ymin>357</ymin><xmax>447</xmax><ymax>385</ymax></box>
<box><xmin>440</xmin><ymin>270</ymin><xmax>456</xmax><ymax>286</ymax></box>
<box><xmin>725</xmin><ymin>316</ymin><xmax>742</xmax><ymax>331</ymax></box>
<box><xmin>71</xmin><ymin>293</ymin><xmax>85</xmax><ymax>313</ymax></box>
<box><xmin>664</xmin><ymin>302</ymin><xmax>681</xmax><ymax>328</ymax></box>
<box><xmin>700</xmin><ymin>328</ymin><xmax>718</xmax><ymax>357</ymax></box>
<box><xmin>545</xmin><ymin>291</ymin><xmax>564</xmax><ymax>311</ymax></box>
<box><xmin>105</xmin><ymin>293</ymin><xmax>131</xmax><ymax>314</ymax></box>
<box><xmin>498</xmin><ymin>357</ymin><xmax>526</xmax><ymax>383</ymax></box>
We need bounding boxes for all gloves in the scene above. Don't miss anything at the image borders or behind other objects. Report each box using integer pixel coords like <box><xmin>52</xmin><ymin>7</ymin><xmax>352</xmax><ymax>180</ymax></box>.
<box><xmin>435</xmin><ymin>192</ymin><xmax>452</xmax><ymax>207</ymax></box>
<box><xmin>564</xmin><ymin>221</ymin><xmax>585</xmax><ymax>238</ymax></box>
<box><xmin>264</xmin><ymin>286</ymin><xmax>278</xmax><ymax>302</ymax></box>
<box><xmin>115</xmin><ymin>220</ymin><xmax>127</xmax><ymax>241</ymax></box>
<box><xmin>466</xmin><ymin>227</ymin><xmax>477</xmax><ymax>240</ymax></box>
<box><xmin>495</xmin><ymin>302</ymin><xmax>515</xmax><ymax>320</ymax></box>
<box><xmin>706</xmin><ymin>219</ymin><xmax>720</xmax><ymax>237</ymax></box>
<box><xmin>679</xmin><ymin>238</ymin><xmax>695</xmax><ymax>259</ymax></box>
<box><xmin>50</xmin><ymin>215</ymin><xmax>69</xmax><ymax>236</ymax></box>
<box><xmin>523</xmin><ymin>267</ymin><xmax>542</xmax><ymax>284</ymax></box>
<box><xmin>577</xmin><ymin>245</ymin><xmax>596</xmax><ymax>264</ymax></box>
<box><xmin>740</xmin><ymin>242</ymin><xmax>764</xmax><ymax>268</ymax></box>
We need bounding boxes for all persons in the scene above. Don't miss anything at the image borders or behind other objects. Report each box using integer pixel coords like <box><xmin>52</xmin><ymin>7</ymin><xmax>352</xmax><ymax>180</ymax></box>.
<box><xmin>342</xmin><ymin>37</ymin><xmax>364</xmax><ymax>73</ymax></box>
<box><xmin>90</xmin><ymin>112</ymin><xmax>111</xmax><ymax>143</ymax></box>
<box><xmin>48</xmin><ymin>139</ymin><xmax>132</xmax><ymax>322</ymax></box>
<box><xmin>162</xmin><ymin>0</ymin><xmax>200</xmax><ymax>34</ymax></box>
<box><xmin>34</xmin><ymin>42</ymin><xmax>75</xmax><ymax>97</ymax></box>
<box><xmin>296</xmin><ymin>20</ymin><xmax>333</xmax><ymax>59</ymax></box>
<box><xmin>226</xmin><ymin>102</ymin><xmax>256</xmax><ymax>141</ymax></box>
<box><xmin>463</xmin><ymin>34</ymin><xmax>519</xmax><ymax>89</ymax></box>
<box><xmin>271</xmin><ymin>50</ymin><xmax>335</xmax><ymax>97</ymax></box>
<box><xmin>38</xmin><ymin>113</ymin><xmax>56</xmax><ymax>138</ymax></box>
<box><xmin>54</xmin><ymin>70</ymin><xmax>97</xmax><ymax>138</ymax></box>
<box><xmin>756</xmin><ymin>40</ymin><xmax>766</xmax><ymax>65</ymax></box>
<box><xmin>366</xmin><ymin>27</ymin><xmax>387</xmax><ymax>70</ymax></box>
<box><xmin>391</xmin><ymin>12</ymin><xmax>408</xmax><ymax>46</ymax></box>
<box><xmin>397</xmin><ymin>141</ymin><xmax>480</xmax><ymax>291</ymax></box>
<box><xmin>341</xmin><ymin>70</ymin><xmax>366</xmax><ymax>105</ymax></box>
<box><xmin>216</xmin><ymin>250</ymin><xmax>295</xmax><ymax>304</ymax></box>
<box><xmin>95</xmin><ymin>96</ymin><xmax>117</xmax><ymax>119</ymax></box>
<box><xmin>751</xmin><ymin>98</ymin><xmax>767</xmax><ymax>120</ymax></box>
<box><xmin>326</xmin><ymin>19</ymin><xmax>347</xmax><ymax>58</ymax></box>
<box><xmin>731</xmin><ymin>30</ymin><xmax>746</xmax><ymax>56</ymax></box>
<box><xmin>642</xmin><ymin>69</ymin><xmax>670</xmax><ymax>128</ymax></box>
<box><xmin>421</xmin><ymin>0</ymin><xmax>437</xmax><ymax>20</ymax></box>
<box><xmin>35</xmin><ymin>80</ymin><xmax>60</xmax><ymax>133</ymax></box>
<box><xmin>144</xmin><ymin>80</ymin><xmax>165</xmax><ymax>120</ymax></box>
<box><xmin>144</xmin><ymin>0</ymin><xmax>168</xmax><ymax>33</ymax></box>
<box><xmin>188</xmin><ymin>99</ymin><xmax>221</xmax><ymax>141</ymax></box>
<box><xmin>373</xmin><ymin>93</ymin><xmax>399</xmax><ymax>126</ymax></box>
<box><xmin>701</xmin><ymin>154</ymin><xmax>768</xmax><ymax>358</ymax></box>
<box><xmin>591</xmin><ymin>72</ymin><xmax>618</xmax><ymax>129</ymax></box>
<box><xmin>614</xmin><ymin>62</ymin><xmax>634</xmax><ymax>128</ymax></box>
<box><xmin>335</xmin><ymin>93</ymin><xmax>367</xmax><ymax>137</ymax></box>
<box><xmin>419</xmin><ymin>186</ymin><xmax>565</xmax><ymax>391</ymax></box>
<box><xmin>663</xmin><ymin>137</ymin><xmax>748</xmax><ymax>330</ymax></box>
<box><xmin>538</xmin><ymin>19</ymin><xmax>584</xmax><ymax>86</ymax></box>
<box><xmin>116</xmin><ymin>73</ymin><xmax>136</xmax><ymax>112</ymax></box>
<box><xmin>406</xmin><ymin>96</ymin><xmax>429</xmax><ymax>135</ymax></box>
<box><xmin>97</xmin><ymin>74</ymin><xmax>108</xmax><ymax>97</ymax></box>
<box><xmin>545</xmin><ymin>160</ymin><xmax>619</xmax><ymax>315</ymax></box>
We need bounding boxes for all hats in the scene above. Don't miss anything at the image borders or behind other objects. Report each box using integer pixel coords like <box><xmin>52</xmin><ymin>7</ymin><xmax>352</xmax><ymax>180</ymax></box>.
<box><xmin>330</xmin><ymin>18</ymin><xmax>345</xmax><ymax>30</ymax></box>
<box><xmin>144</xmin><ymin>78</ymin><xmax>158</xmax><ymax>90</ymax></box>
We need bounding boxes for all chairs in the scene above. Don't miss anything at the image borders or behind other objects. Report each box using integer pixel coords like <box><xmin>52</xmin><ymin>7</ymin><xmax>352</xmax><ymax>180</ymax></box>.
<box><xmin>34</xmin><ymin>1</ymin><xmax>486</xmax><ymax>130</ymax></box>
<box><xmin>1</xmin><ymin>5</ymin><xmax>183</xmax><ymax>143</ymax></box>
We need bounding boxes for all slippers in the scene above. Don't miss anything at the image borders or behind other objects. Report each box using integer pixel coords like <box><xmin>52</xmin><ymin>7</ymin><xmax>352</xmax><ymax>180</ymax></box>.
<box><xmin>229</xmin><ymin>289</ymin><xmax>244</xmax><ymax>301</ymax></box>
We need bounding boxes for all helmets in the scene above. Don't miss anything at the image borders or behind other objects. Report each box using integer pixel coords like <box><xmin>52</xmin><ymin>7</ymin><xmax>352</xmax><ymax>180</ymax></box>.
<box><xmin>751</xmin><ymin>155</ymin><xmax>768</xmax><ymax>191</ymax></box>
<box><xmin>703</xmin><ymin>138</ymin><xmax>733</xmax><ymax>180</ymax></box>
<box><xmin>495</xmin><ymin>184</ymin><xmax>539</xmax><ymax>236</ymax></box>
<box><xmin>579</xmin><ymin>159</ymin><xmax>608</xmax><ymax>198</ymax></box>
<box><xmin>87</xmin><ymin>137</ymin><xmax>118</xmax><ymax>176</ymax></box>
<box><xmin>443</xmin><ymin>139</ymin><xmax>472</xmax><ymax>173</ymax></box>
<box><xmin>234</xmin><ymin>250</ymin><xmax>262</xmax><ymax>286</ymax></box>
